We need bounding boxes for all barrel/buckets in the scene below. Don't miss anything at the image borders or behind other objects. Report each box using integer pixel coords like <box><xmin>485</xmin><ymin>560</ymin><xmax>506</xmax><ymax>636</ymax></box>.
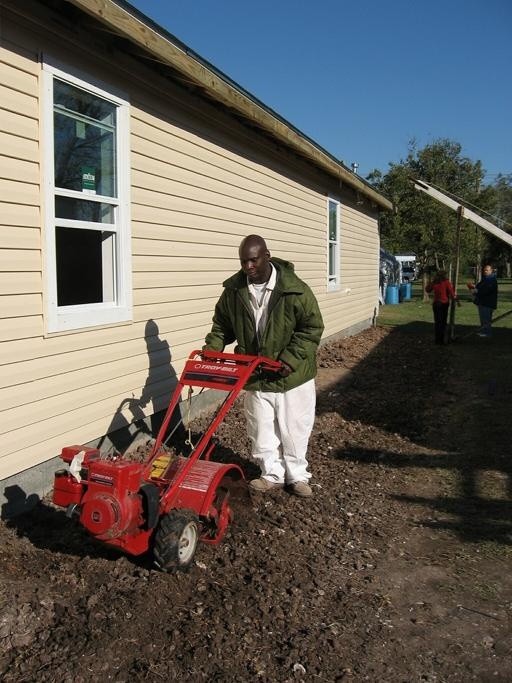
<box><xmin>400</xmin><ymin>283</ymin><xmax>412</xmax><ymax>300</ymax></box>
<box><xmin>386</xmin><ymin>284</ymin><xmax>399</xmax><ymax>304</ymax></box>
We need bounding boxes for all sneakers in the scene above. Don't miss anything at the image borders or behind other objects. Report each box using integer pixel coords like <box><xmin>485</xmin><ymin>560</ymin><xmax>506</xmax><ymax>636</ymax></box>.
<box><xmin>248</xmin><ymin>477</ymin><xmax>287</xmax><ymax>491</ymax></box>
<box><xmin>289</xmin><ymin>481</ymin><xmax>313</xmax><ymax>497</ymax></box>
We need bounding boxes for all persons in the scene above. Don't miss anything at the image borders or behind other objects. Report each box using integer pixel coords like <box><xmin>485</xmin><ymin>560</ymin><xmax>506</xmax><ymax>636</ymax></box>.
<box><xmin>425</xmin><ymin>268</ymin><xmax>462</xmax><ymax>345</ymax></box>
<box><xmin>466</xmin><ymin>264</ymin><xmax>498</xmax><ymax>337</ymax></box>
<box><xmin>202</xmin><ymin>234</ymin><xmax>325</xmax><ymax>498</ymax></box>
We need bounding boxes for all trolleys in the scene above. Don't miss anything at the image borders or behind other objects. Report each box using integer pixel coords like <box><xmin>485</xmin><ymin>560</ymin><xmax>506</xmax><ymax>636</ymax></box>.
<box><xmin>49</xmin><ymin>348</ymin><xmax>282</xmax><ymax>572</ymax></box>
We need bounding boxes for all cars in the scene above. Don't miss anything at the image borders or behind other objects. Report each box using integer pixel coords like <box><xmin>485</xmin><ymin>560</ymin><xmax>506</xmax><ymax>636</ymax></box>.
<box><xmin>402</xmin><ymin>266</ymin><xmax>416</xmax><ymax>280</ymax></box>
